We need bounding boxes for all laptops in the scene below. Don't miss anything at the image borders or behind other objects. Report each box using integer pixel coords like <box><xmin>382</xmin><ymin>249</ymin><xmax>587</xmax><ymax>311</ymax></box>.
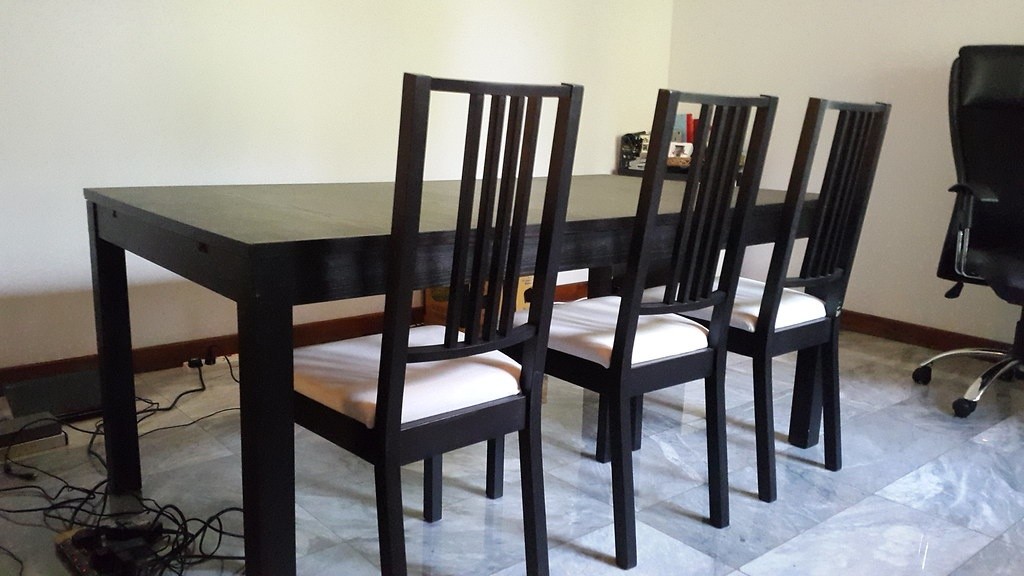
<box><xmin>5</xmin><ymin>369</ymin><xmax>104</xmax><ymax>426</ymax></box>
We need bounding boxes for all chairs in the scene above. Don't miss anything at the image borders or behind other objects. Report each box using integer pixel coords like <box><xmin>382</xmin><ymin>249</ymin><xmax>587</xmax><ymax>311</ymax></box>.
<box><xmin>292</xmin><ymin>72</ymin><xmax>583</xmax><ymax>575</ymax></box>
<box><xmin>482</xmin><ymin>88</ymin><xmax>779</xmax><ymax>569</ymax></box>
<box><xmin>911</xmin><ymin>44</ymin><xmax>1024</xmax><ymax>418</ymax></box>
<box><xmin>634</xmin><ymin>99</ymin><xmax>892</xmax><ymax>503</ymax></box>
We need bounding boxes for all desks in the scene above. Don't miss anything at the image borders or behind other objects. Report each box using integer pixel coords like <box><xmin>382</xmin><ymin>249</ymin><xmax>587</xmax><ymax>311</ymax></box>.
<box><xmin>83</xmin><ymin>174</ymin><xmax>819</xmax><ymax>576</ymax></box>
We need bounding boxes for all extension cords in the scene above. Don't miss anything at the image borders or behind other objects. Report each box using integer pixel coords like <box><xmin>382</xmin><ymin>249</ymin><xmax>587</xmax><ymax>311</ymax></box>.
<box><xmin>184</xmin><ymin>354</ymin><xmax>239</xmax><ymax>374</ymax></box>
<box><xmin>56</xmin><ymin>537</ymin><xmax>99</xmax><ymax>576</ymax></box>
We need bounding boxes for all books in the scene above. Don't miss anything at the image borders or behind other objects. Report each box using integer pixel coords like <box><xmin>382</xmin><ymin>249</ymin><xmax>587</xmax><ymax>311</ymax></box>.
<box><xmin>672</xmin><ymin>113</ymin><xmax>694</xmax><ymax>143</ymax></box>
